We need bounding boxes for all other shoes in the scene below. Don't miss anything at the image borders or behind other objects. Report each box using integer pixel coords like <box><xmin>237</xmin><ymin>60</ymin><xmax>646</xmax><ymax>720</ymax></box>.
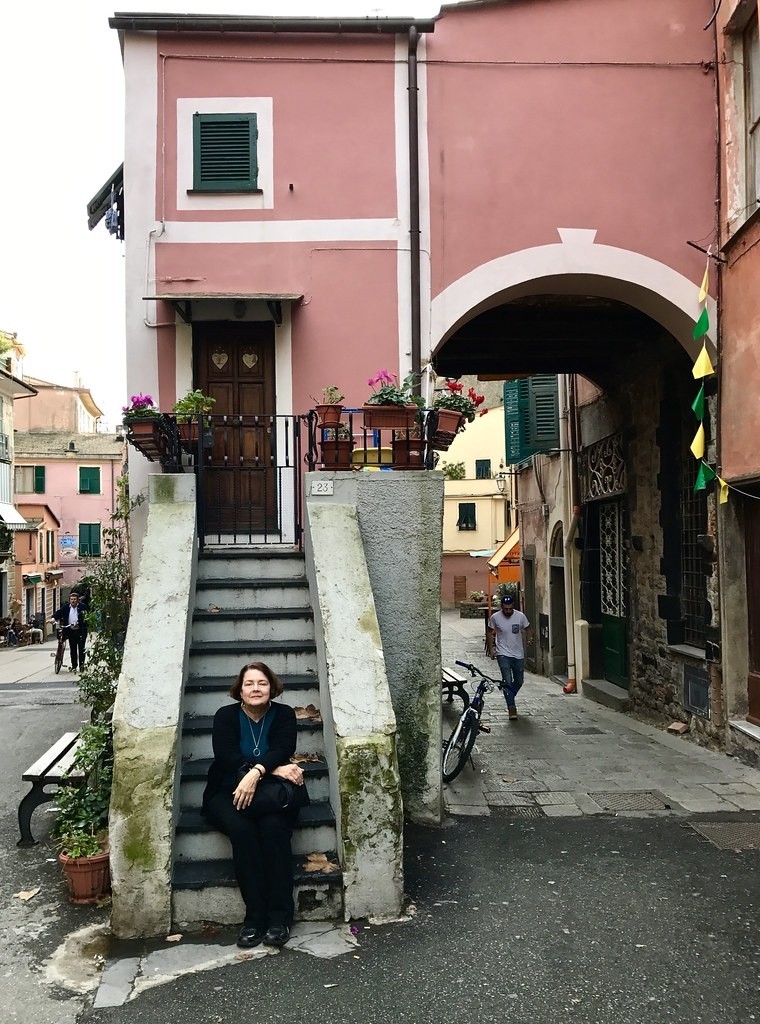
<box><xmin>13</xmin><ymin>644</ymin><xmax>17</xmax><ymax>647</ymax></box>
<box><xmin>9</xmin><ymin>642</ymin><xmax>13</xmax><ymax>645</ymax></box>
<box><xmin>508</xmin><ymin>705</ymin><xmax>517</xmax><ymax>720</ymax></box>
<box><xmin>71</xmin><ymin>665</ymin><xmax>77</xmax><ymax>671</ymax></box>
<box><xmin>80</xmin><ymin>668</ymin><xmax>84</xmax><ymax>672</ymax></box>
<box><xmin>40</xmin><ymin>641</ymin><xmax>43</xmax><ymax>643</ymax></box>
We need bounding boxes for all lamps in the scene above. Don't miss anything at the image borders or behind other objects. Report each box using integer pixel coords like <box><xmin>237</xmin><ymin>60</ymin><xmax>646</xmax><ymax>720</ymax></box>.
<box><xmin>496</xmin><ymin>472</ymin><xmax>522</xmax><ymax>492</ymax></box>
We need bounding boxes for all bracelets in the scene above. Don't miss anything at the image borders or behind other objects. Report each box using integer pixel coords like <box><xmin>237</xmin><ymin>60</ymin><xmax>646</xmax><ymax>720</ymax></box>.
<box><xmin>253</xmin><ymin>767</ymin><xmax>263</xmax><ymax>780</ymax></box>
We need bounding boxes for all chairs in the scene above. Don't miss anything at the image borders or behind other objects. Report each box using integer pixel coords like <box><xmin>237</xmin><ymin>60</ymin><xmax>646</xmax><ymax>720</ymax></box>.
<box><xmin>0</xmin><ymin>625</ymin><xmax>43</xmax><ymax>647</ymax></box>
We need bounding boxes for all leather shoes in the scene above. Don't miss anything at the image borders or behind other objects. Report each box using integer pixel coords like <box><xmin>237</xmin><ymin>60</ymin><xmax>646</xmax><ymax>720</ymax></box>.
<box><xmin>237</xmin><ymin>924</ymin><xmax>265</xmax><ymax>947</ymax></box>
<box><xmin>263</xmin><ymin>926</ymin><xmax>290</xmax><ymax>946</ymax></box>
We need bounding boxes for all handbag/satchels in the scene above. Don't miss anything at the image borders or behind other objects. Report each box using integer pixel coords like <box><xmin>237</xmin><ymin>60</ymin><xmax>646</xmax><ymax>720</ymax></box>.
<box><xmin>237</xmin><ymin>762</ymin><xmax>298</xmax><ymax>809</ymax></box>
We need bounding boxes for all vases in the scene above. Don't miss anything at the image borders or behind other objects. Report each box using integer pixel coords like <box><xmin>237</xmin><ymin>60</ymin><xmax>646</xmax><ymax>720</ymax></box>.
<box><xmin>362</xmin><ymin>401</ymin><xmax>418</xmax><ymax>429</ymax></box>
<box><xmin>433</xmin><ymin>409</ymin><xmax>462</xmax><ymax>451</ymax></box>
<box><xmin>130</xmin><ymin>422</ymin><xmax>162</xmax><ymax>448</ymax></box>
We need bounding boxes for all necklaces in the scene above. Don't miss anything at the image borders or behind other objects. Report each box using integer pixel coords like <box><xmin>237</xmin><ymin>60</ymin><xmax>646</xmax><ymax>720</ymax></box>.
<box><xmin>242</xmin><ymin>708</ymin><xmax>266</xmax><ymax>757</ymax></box>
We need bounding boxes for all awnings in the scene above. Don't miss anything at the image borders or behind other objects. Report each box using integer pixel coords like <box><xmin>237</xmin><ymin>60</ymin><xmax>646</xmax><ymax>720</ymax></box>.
<box><xmin>0</xmin><ymin>503</ymin><xmax>28</xmax><ymax>530</ymax></box>
<box><xmin>485</xmin><ymin>525</ymin><xmax>519</xmax><ymax>580</ymax></box>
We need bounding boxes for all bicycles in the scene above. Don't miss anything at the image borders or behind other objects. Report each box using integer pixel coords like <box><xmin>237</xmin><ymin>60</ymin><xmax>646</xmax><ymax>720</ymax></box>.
<box><xmin>48</xmin><ymin>621</ymin><xmax>76</xmax><ymax>675</ymax></box>
<box><xmin>438</xmin><ymin>658</ymin><xmax>517</xmax><ymax>785</ymax></box>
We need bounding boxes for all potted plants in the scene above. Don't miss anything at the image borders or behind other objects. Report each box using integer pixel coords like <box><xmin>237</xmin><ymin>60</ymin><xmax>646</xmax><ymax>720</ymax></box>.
<box><xmin>309</xmin><ymin>385</ymin><xmax>345</xmax><ymax>422</ymax></box>
<box><xmin>467</xmin><ymin>590</ymin><xmax>484</xmax><ymax>602</ymax></box>
<box><xmin>390</xmin><ymin>395</ymin><xmax>428</xmax><ymax>467</ymax></box>
<box><xmin>173</xmin><ymin>388</ymin><xmax>216</xmax><ymax>446</ymax></box>
<box><xmin>59</xmin><ymin>829</ymin><xmax>111</xmax><ymax>904</ymax></box>
<box><xmin>317</xmin><ymin>421</ymin><xmax>358</xmax><ymax>467</ymax></box>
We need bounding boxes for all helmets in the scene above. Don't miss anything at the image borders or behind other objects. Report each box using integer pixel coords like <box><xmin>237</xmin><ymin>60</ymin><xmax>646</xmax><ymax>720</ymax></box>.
<box><xmin>501</xmin><ymin>595</ymin><xmax>513</xmax><ymax>605</ymax></box>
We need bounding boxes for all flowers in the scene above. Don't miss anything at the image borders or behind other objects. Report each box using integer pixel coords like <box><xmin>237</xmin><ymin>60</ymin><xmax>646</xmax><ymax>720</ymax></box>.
<box><xmin>121</xmin><ymin>393</ymin><xmax>160</xmax><ymax>425</ymax></box>
<box><xmin>366</xmin><ymin>370</ymin><xmax>421</xmax><ymax>408</ymax></box>
<box><xmin>434</xmin><ymin>380</ymin><xmax>488</xmax><ymax>433</ymax></box>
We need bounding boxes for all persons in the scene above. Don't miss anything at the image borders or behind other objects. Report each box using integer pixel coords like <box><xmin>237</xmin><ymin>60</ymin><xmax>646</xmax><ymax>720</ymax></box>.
<box><xmin>0</xmin><ymin>614</ymin><xmax>56</xmax><ymax>647</ymax></box>
<box><xmin>200</xmin><ymin>662</ymin><xmax>312</xmax><ymax>949</ymax></box>
<box><xmin>50</xmin><ymin>593</ymin><xmax>89</xmax><ymax>672</ymax></box>
<box><xmin>487</xmin><ymin>595</ymin><xmax>535</xmax><ymax>721</ymax></box>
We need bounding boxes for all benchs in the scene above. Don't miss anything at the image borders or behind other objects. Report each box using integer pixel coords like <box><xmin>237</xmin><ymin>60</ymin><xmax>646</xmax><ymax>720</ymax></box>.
<box><xmin>17</xmin><ymin>702</ymin><xmax>115</xmax><ymax>849</ymax></box>
<box><xmin>442</xmin><ymin>667</ymin><xmax>469</xmax><ymax>716</ymax></box>
